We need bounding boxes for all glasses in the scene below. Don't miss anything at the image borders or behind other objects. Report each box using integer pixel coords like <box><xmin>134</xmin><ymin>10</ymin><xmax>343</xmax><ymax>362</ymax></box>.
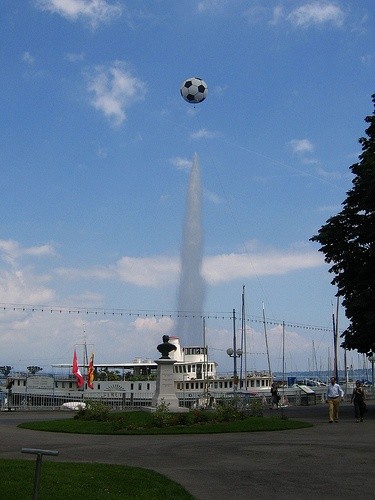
<box><xmin>356</xmin><ymin>382</ymin><xmax>359</xmax><ymax>384</ymax></box>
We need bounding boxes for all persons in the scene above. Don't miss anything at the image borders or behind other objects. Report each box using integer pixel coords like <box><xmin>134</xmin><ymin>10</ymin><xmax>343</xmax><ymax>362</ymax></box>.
<box><xmin>350</xmin><ymin>380</ymin><xmax>365</xmax><ymax>423</ymax></box>
<box><xmin>325</xmin><ymin>377</ymin><xmax>344</xmax><ymax>423</ymax></box>
<box><xmin>157</xmin><ymin>335</ymin><xmax>177</xmax><ymax>359</ymax></box>
<box><xmin>270</xmin><ymin>383</ymin><xmax>280</xmax><ymax>409</ymax></box>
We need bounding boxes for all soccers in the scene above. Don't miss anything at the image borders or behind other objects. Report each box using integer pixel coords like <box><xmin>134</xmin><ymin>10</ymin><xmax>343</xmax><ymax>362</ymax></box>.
<box><xmin>180</xmin><ymin>78</ymin><xmax>208</xmax><ymax>104</ymax></box>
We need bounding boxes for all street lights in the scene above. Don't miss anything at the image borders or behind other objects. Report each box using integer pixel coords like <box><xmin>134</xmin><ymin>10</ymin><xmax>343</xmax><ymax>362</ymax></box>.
<box><xmin>226</xmin><ymin>309</ymin><xmax>244</xmax><ymax>397</ymax></box>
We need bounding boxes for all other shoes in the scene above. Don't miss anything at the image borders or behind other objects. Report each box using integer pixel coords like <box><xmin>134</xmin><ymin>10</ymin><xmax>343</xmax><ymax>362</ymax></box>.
<box><xmin>329</xmin><ymin>420</ymin><xmax>338</xmax><ymax>424</ymax></box>
<box><xmin>356</xmin><ymin>419</ymin><xmax>364</xmax><ymax>423</ymax></box>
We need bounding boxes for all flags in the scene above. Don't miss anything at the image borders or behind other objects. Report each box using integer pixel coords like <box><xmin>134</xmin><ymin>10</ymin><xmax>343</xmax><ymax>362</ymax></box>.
<box><xmin>87</xmin><ymin>352</ymin><xmax>95</xmax><ymax>389</ymax></box>
<box><xmin>72</xmin><ymin>348</ymin><xmax>85</xmax><ymax>388</ymax></box>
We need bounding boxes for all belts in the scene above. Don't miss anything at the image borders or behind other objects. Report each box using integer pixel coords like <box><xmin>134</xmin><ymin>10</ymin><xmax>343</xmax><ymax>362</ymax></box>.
<box><xmin>329</xmin><ymin>395</ymin><xmax>340</xmax><ymax>399</ymax></box>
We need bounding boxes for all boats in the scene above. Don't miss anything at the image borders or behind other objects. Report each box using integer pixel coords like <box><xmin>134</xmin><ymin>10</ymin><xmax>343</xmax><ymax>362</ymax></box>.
<box><xmin>0</xmin><ymin>283</ymin><xmax>375</xmax><ymax>407</ymax></box>
